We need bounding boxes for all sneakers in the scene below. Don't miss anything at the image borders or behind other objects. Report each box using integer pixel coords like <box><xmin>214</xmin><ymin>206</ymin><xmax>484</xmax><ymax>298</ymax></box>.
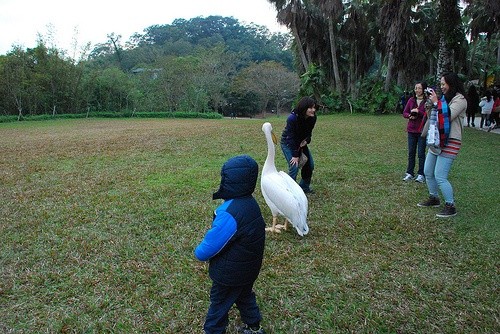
<box><xmin>415</xmin><ymin>175</ymin><xmax>425</xmax><ymax>183</ymax></box>
<box><xmin>436</xmin><ymin>204</ymin><xmax>457</xmax><ymax>217</ymax></box>
<box><xmin>240</xmin><ymin>324</ymin><xmax>264</xmax><ymax>334</ymax></box>
<box><xmin>403</xmin><ymin>173</ymin><xmax>413</xmax><ymax>181</ymax></box>
<box><xmin>416</xmin><ymin>197</ymin><xmax>441</xmax><ymax>207</ymax></box>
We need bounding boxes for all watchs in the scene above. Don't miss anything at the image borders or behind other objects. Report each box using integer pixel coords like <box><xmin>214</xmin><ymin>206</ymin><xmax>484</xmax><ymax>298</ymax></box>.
<box><xmin>434</xmin><ymin>101</ymin><xmax>438</xmax><ymax>105</ymax></box>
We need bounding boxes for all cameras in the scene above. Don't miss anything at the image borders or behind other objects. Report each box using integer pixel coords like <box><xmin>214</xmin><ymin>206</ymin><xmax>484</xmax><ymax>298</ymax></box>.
<box><xmin>425</xmin><ymin>89</ymin><xmax>432</xmax><ymax>96</ymax></box>
<box><xmin>411</xmin><ymin>112</ymin><xmax>417</xmax><ymax>116</ymax></box>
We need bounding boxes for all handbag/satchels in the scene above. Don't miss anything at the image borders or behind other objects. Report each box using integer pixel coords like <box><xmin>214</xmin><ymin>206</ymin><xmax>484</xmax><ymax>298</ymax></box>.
<box><xmin>427</xmin><ymin>110</ymin><xmax>440</xmax><ymax>148</ymax></box>
<box><xmin>298</xmin><ymin>148</ymin><xmax>308</xmax><ymax>169</ymax></box>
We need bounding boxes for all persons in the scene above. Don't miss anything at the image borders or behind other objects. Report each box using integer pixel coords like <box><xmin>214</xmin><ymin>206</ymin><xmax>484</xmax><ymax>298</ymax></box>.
<box><xmin>194</xmin><ymin>156</ymin><xmax>266</xmax><ymax>334</ymax></box>
<box><xmin>403</xmin><ymin>80</ymin><xmax>430</xmax><ymax>182</ymax></box>
<box><xmin>280</xmin><ymin>97</ymin><xmax>317</xmax><ymax>194</ymax></box>
<box><xmin>466</xmin><ymin>84</ymin><xmax>500</xmax><ymax>130</ymax></box>
<box><xmin>417</xmin><ymin>73</ymin><xmax>467</xmax><ymax>218</ymax></box>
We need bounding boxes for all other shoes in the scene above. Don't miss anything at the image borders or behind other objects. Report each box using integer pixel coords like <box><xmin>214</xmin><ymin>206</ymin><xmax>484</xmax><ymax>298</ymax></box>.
<box><xmin>480</xmin><ymin>128</ymin><xmax>483</xmax><ymax>131</ymax></box>
<box><xmin>472</xmin><ymin>124</ymin><xmax>475</xmax><ymax>127</ymax></box>
<box><xmin>467</xmin><ymin>125</ymin><xmax>470</xmax><ymax>127</ymax></box>
<box><xmin>488</xmin><ymin>126</ymin><xmax>491</xmax><ymax>132</ymax></box>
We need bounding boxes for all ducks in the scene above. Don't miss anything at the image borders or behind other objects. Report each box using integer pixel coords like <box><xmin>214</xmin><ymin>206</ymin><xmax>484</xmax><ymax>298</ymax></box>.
<box><xmin>259</xmin><ymin>122</ymin><xmax>311</xmax><ymax>237</ymax></box>
